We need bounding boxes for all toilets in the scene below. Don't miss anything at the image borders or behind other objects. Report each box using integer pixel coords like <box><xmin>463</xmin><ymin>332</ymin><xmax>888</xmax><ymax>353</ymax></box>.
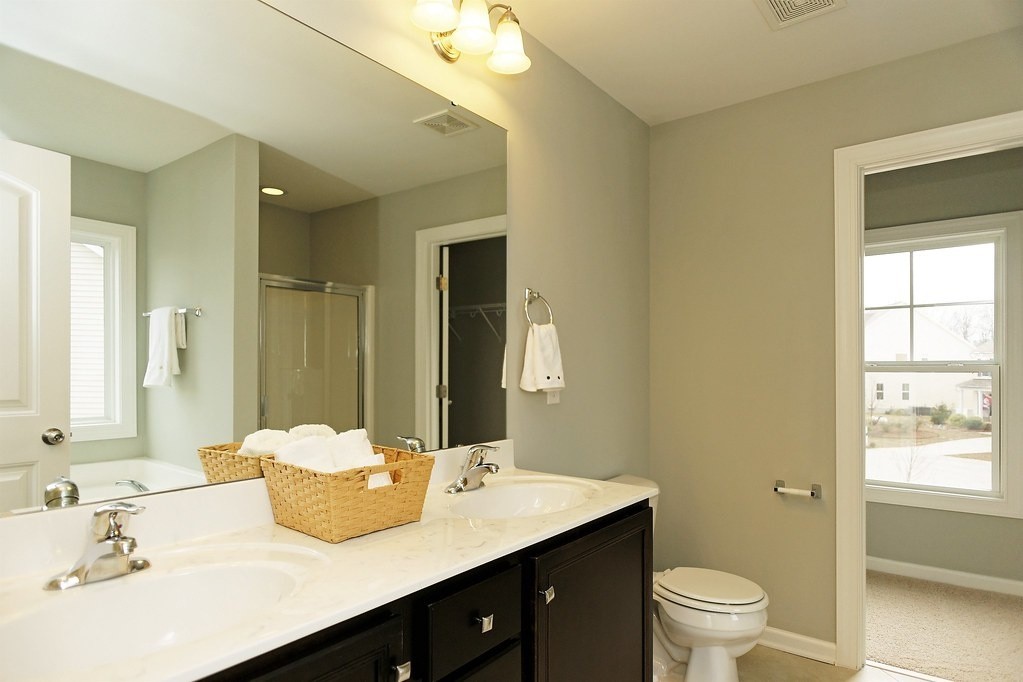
<box><xmin>603</xmin><ymin>472</ymin><xmax>770</xmax><ymax>682</ymax></box>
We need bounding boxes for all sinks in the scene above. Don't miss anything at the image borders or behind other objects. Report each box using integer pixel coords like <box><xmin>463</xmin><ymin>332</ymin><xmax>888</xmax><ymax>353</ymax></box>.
<box><xmin>2</xmin><ymin>540</ymin><xmax>332</xmax><ymax>681</ymax></box>
<box><xmin>415</xmin><ymin>467</ymin><xmax>604</xmax><ymax>522</ymax></box>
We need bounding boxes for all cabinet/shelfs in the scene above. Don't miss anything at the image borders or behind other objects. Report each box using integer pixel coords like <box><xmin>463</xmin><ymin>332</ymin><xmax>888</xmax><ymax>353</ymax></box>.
<box><xmin>252</xmin><ymin>507</ymin><xmax>654</xmax><ymax>682</ymax></box>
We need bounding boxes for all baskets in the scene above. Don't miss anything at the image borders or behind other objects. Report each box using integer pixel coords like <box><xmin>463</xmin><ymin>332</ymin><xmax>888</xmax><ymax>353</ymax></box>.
<box><xmin>259</xmin><ymin>444</ymin><xmax>435</xmax><ymax>544</ymax></box>
<box><xmin>196</xmin><ymin>442</ymin><xmax>273</xmax><ymax>484</ymax></box>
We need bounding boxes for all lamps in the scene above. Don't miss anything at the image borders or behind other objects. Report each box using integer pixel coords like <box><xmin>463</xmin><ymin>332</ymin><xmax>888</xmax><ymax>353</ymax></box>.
<box><xmin>430</xmin><ymin>5</ymin><xmax>532</xmax><ymax>75</ymax></box>
<box><xmin>450</xmin><ymin>0</ymin><xmax>500</xmax><ymax>55</ymax></box>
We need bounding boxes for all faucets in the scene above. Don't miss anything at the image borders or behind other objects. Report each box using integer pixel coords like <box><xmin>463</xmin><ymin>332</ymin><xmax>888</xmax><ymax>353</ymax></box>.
<box><xmin>443</xmin><ymin>444</ymin><xmax>503</xmax><ymax>494</ymax></box>
<box><xmin>114</xmin><ymin>478</ymin><xmax>151</xmax><ymax>493</ymax></box>
<box><xmin>40</xmin><ymin>502</ymin><xmax>151</xmax><ymax>592</ymax></box>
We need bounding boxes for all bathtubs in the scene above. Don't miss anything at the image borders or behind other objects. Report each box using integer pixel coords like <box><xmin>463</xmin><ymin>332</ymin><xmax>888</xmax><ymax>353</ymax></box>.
<box><xmin>70</xmin><ymin>455</ymin><xmax>209</xmax><ymax>504</ymax></box>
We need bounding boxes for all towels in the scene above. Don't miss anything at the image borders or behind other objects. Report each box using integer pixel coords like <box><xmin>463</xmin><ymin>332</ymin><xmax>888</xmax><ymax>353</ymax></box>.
<box><xmin>142</xmin><ymin>305</ymin><xmax>188</xmax><ymax>391</ymax></box>
<box><xmin>519</xmin><ymin>323</ymin><xmax>567</xmax><ymax>406</ymax></box>
<box><xmin>236</xmin><ymin>428</ymin><xmax>289</xmax><ymax>457</ymax></box>
<box><xmin>273</xmin><ymin>427</ymin><xmax>394</xmax><ymax>491</ymax></box>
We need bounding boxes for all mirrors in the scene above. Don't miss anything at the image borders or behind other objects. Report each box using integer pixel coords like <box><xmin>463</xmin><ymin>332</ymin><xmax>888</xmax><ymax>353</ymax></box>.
<box><xmin>0</xmin><ymin>0</ymin><xmax>506</xmax><ymax>519</ymax></box>
